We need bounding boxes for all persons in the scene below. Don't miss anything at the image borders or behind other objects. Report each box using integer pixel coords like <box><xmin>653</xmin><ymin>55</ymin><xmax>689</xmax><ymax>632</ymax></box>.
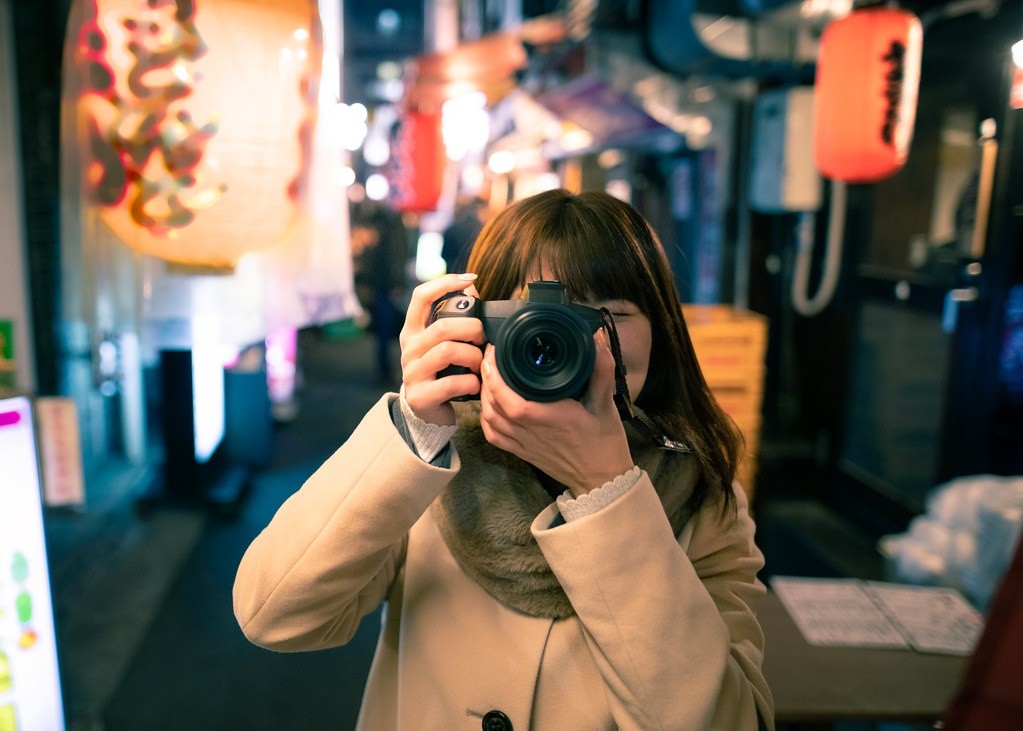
<box><xmin>232</xmin><ymin>189</ymin><xmax>774</xmax><ymax>731</ymax></box>
<box><xmin>355</xmin><ymin>200</ymin><xmax>407</xmax><ymax>385</ymax></box>
<box><xmin>442</xmin><ymin>203</ymin><xmax>484</xmax><ymax>297</ymax></box>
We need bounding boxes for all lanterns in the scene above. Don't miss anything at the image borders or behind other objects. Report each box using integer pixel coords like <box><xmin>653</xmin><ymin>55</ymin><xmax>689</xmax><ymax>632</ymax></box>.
<box><xmin>59</xmin><ymin>0</ymin><xmax>327</xmax><ymax>272</ymax></box>
<box><xmin>812</xmin><ymin>9</ymin><xmax>923</xmax><ymax>183</ymax></box>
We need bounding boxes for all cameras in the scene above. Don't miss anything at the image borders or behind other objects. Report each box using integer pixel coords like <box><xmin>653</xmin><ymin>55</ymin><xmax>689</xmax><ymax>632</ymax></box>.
<box><xmin>433</xmin><ymin>281</ymin><xmax>605</xmax><ymax>402</ymax></box>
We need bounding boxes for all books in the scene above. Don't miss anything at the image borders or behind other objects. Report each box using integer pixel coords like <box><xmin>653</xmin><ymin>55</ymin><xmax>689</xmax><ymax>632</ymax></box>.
<box><xmin>770</xmin><ymin>576</ymin><xmax>987</xmax><ymax>654</ymax></box>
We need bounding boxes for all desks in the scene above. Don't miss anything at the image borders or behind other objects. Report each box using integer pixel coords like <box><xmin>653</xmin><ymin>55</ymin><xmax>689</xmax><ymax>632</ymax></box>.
<box><xmin>748</xmin><ymin>585</ymin><xmax>985</xmax><ymax>719</ymax></box>
